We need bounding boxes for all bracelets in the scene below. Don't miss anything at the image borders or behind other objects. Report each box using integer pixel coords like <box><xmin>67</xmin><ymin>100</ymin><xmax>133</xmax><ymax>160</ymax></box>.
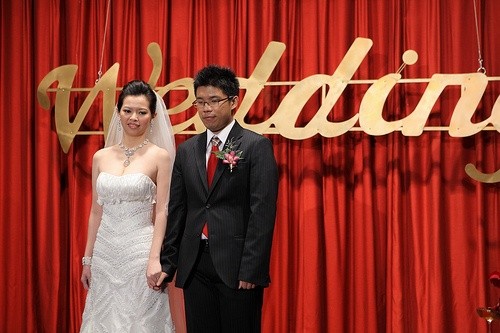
<box><xmin>82</xmin><ymin>255</ymin><xmax>92</xmax><ymax>265</ymax></box>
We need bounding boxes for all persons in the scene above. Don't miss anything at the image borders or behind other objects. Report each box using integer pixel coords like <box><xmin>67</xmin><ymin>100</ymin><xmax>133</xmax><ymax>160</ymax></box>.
<box><xmin>81</xmin><ymin>79</ymin><xmax>171</xmax><ymax>333</ymax></box>
<box><xmin>146</xmin><ymin>63</ymin><xmax>280</xmax><ymax>333</ymax></box>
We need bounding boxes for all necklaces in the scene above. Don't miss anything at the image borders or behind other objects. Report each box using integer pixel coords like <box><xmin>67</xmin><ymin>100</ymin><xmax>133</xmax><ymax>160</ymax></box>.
<box><xmin>117</xmin><ymin>137</ymin><xmax>150</xmax><ymax>166</ymax></box>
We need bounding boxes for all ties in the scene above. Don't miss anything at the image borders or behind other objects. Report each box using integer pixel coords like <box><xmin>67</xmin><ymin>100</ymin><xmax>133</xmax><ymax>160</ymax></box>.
<box><xmin>202</xmin><ymin>136</ymin><xmax>222</xmax><ymax>238</ymax></box>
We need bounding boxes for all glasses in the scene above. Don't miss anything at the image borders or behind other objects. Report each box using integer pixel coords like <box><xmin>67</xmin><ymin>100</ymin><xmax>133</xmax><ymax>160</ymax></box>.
<box><xmin>192</xmin><ymin>95</ymin><xmax>233</xmax><ymax>107</ymax></box>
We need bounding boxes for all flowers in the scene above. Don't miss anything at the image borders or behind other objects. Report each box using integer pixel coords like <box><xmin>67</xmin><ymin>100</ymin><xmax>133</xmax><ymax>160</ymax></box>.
<box><xmin>210</xmin><ymin>137</ymin><xmax>244</xmax><ymax>172</ymax></box>
